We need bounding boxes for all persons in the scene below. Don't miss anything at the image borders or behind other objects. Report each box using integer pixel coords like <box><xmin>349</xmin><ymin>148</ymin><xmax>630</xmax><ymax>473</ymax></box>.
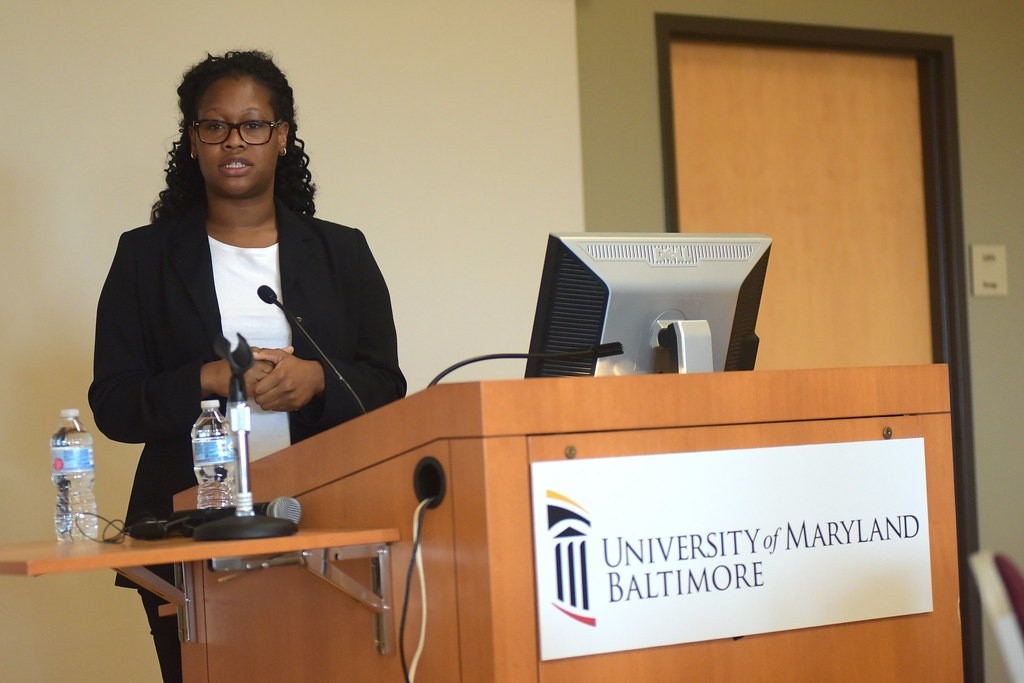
<box><xmin>87</xmin><ymin>50</ymin><xmax>407</xmax><ymax>683</ymax></box>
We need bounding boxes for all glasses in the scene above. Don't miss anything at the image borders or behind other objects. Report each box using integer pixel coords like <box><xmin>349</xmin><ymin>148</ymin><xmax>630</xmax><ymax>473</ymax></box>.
<box><xmin>192</xmin><ymin>117</ymin><xmax>284</xmax><ymax>146</ymax></box>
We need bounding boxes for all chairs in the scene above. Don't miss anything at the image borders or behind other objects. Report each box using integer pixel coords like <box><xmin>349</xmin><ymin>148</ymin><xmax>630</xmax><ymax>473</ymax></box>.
<box><xmin>967</xmin><ymin>550</ymin><xmax>1024</xmax><ymax>683</ymax></box>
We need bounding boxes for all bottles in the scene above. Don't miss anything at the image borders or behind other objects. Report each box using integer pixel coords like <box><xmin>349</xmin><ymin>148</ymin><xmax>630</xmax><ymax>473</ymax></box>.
<box><xmin>190</xmin><ymin>400</ymin><xmax>236</xmax><ymax>509</ymax></box>
<box><xmin>49</xmin><ymin>409</ymin><xmax>97</xmax><ymax>541</ymax></box>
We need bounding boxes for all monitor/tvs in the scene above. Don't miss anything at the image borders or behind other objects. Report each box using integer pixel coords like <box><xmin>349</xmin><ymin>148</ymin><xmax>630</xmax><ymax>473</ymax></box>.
<box><xmin>525</xmin><ymin>233</ymin><xmax>773</xmax><ymax>378</ymax></box>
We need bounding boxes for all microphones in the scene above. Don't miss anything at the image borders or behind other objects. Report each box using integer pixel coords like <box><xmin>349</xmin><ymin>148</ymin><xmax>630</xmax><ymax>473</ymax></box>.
<box><xmin>169</xmin><ymin>496</ymin><xmax>301</xmax><ymax>526</ymax></box>
<box><xmin>258</xmin><ymin>285</ymin><xmax>367</xmax><ymax>416</ymax></box>
